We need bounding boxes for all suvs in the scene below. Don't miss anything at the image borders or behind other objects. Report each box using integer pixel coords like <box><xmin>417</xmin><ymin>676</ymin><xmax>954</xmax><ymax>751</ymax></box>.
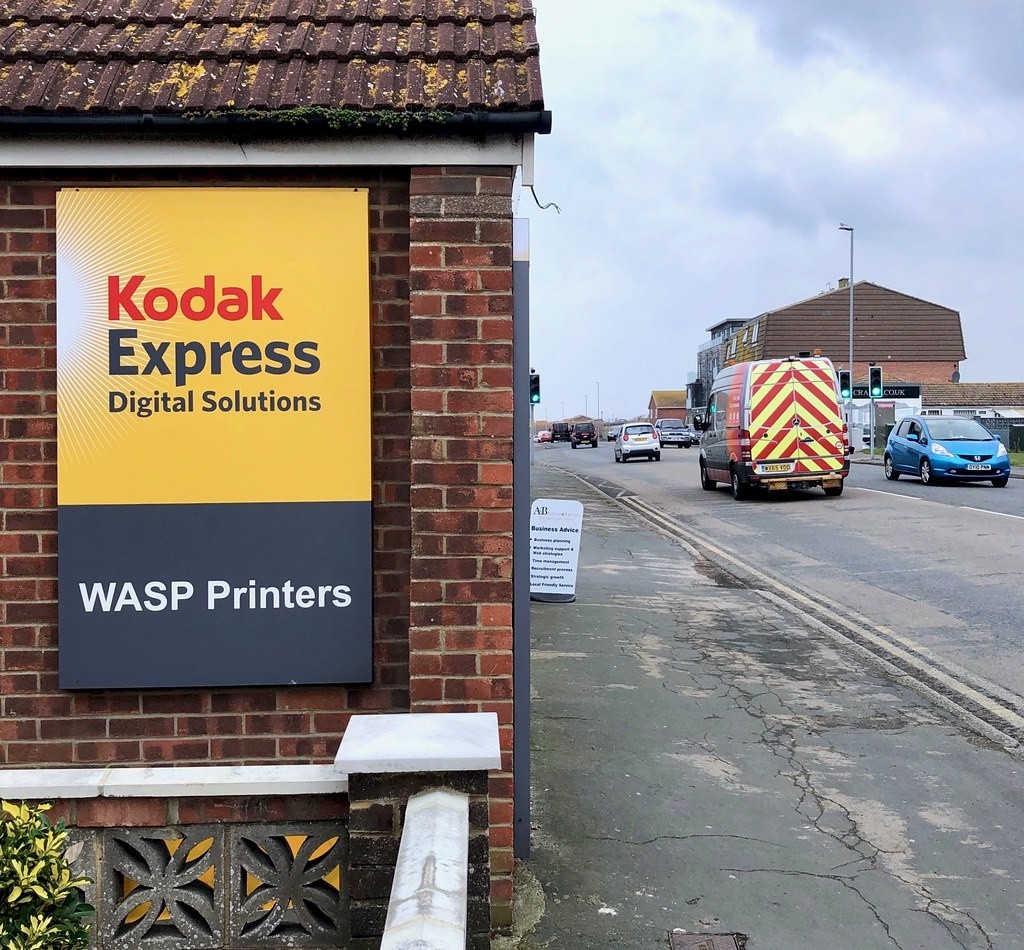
<box><xmin>613</xmin><ymin>422</ymin><xmax>661</xmax><ymax>463</ymax></box>
<box><xmin>571</xmin><ymin>421</ymin><xmax>599</xmax><ymax>449</ymax></box>
<box><xmin>654</xmin><ymin>417</ymin><xmax>693</xmax><ymax>449</ymax></box>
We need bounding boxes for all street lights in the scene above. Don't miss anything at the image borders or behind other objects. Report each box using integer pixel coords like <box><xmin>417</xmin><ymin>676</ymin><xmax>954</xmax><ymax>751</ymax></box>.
<box><xmin>838</xmin><ymin>220</ymin><xmax>858</xmax><ymax>455</ymax></box>
<box><xmin>595</xmin><ymin>381</ymin><xmax>600</xmax><ymax>446</ymax></box>
<box><xmin>584</xmin><ymin>395</ymin><xmax>587</xmax><ymax>423</ymax></box>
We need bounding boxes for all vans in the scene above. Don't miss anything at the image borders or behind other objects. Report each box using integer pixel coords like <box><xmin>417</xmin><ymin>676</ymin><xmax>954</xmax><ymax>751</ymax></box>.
<box><xmin>692</xmin><ymin>348</ymin><xmax>855</xmax><ymax>501</ymax></box>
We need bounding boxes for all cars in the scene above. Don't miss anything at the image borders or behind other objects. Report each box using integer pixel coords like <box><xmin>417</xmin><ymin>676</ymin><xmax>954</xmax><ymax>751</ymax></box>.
<box><xmin>606</xmin><ymin>426</ymin><xmax>621</xmax><ymax>442</ymax></box>
<box><xmin>883</xmin><ymin>412</ymin><xmax>1012</xmax><ymax>488</ymax></box>
<box><xmin>604</xmin><ymin>417</ymin><xmax>628</xmax><ymax>426</ymax></box>
<box><xmin>533</xmin><ymin>421</ymin><xmax>571</xmax><ymax>444</ymax></box>
<box><xmin>686</xmin><ymin>423</ymin><xmax>703</xmax><ymax>446</ymax></box>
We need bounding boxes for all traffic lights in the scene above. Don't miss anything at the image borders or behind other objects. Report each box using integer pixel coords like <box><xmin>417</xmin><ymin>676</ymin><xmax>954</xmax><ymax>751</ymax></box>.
<box><xmin>529</xmin><ymin>373</ymin><xmax>541</xmax><ymax>405</ymax></box>
<box><xmin>838</xmin><ymin>369</ymin><xmax>850</xmax><ymax>399</ymax></box>
<box><xmin>869</xmin><ymin>366</ymin><xmax>883</xmax><ymax>398</ymax></box>
